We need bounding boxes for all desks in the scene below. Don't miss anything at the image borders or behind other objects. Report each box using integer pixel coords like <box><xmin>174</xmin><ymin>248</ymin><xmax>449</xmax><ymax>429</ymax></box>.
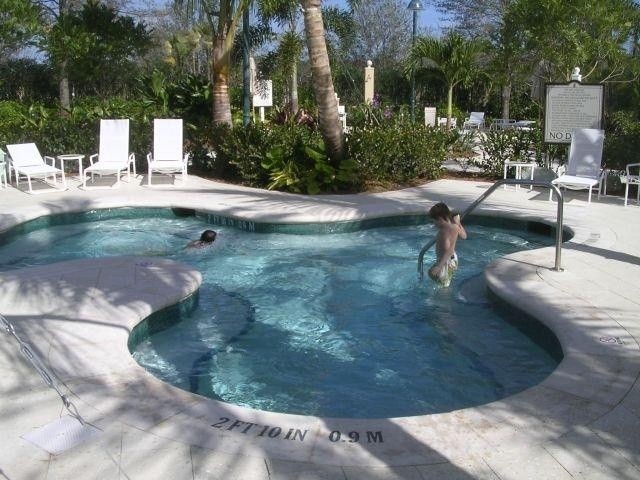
<box><xmin>57</xmin><ymin>154</ymin><xmax>86</xmax><ymax>186</ymax></box>
<box><xmin>503</xmin><ymin>162</ymin><xmax>535</xmax><ymax>191</ymax></box>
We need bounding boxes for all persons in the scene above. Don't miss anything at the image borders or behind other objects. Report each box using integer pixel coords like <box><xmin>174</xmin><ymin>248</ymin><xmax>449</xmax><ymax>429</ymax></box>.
<box><xmin>186</xmin><ymin>230</ymin><xmax>216</xmax><ymax>249</ymax></box>
<box><xmin>428</xmin><ymin>202</ymin><xmax>467</xmax><ymax>295</ymax></box>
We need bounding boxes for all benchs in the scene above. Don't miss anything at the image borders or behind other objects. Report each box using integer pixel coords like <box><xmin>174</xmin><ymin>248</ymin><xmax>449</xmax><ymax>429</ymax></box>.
<box><xmin>438</xmin><ymin>117</ymin><xmax>457</xmax><ymax>129</ymax></box>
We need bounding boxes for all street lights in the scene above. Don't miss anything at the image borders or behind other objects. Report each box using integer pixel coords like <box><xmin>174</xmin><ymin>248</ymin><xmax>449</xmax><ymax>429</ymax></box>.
<box><xmin>406</xmin><ymin>0</ymin><xmax>424</xmax><ymax>125</ymax></box>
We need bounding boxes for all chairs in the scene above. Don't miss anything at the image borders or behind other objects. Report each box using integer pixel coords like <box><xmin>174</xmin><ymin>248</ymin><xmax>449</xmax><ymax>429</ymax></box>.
<box><xmin>620</xmin><ymin>163</ymin><xmax>640</xmax><ymax>207</ymax></box>
<box><xmin>549</xmin><ymin>129</ymin><xmax>607</xmax><ymax>203</ymax></box>
<box><xmin>6</xmin><ymin>143</ymin><xmax>65</xmax><ymax>194</ymax></box>
<box><xmin>82</xmin><ymin>119</ymin><xmax>189</xmax><ymax>190</ymax></box>
<box><xmin>463</xmin><ymin>112</ymin><xmax>537</xmax><ymax>131</ymax></box>
